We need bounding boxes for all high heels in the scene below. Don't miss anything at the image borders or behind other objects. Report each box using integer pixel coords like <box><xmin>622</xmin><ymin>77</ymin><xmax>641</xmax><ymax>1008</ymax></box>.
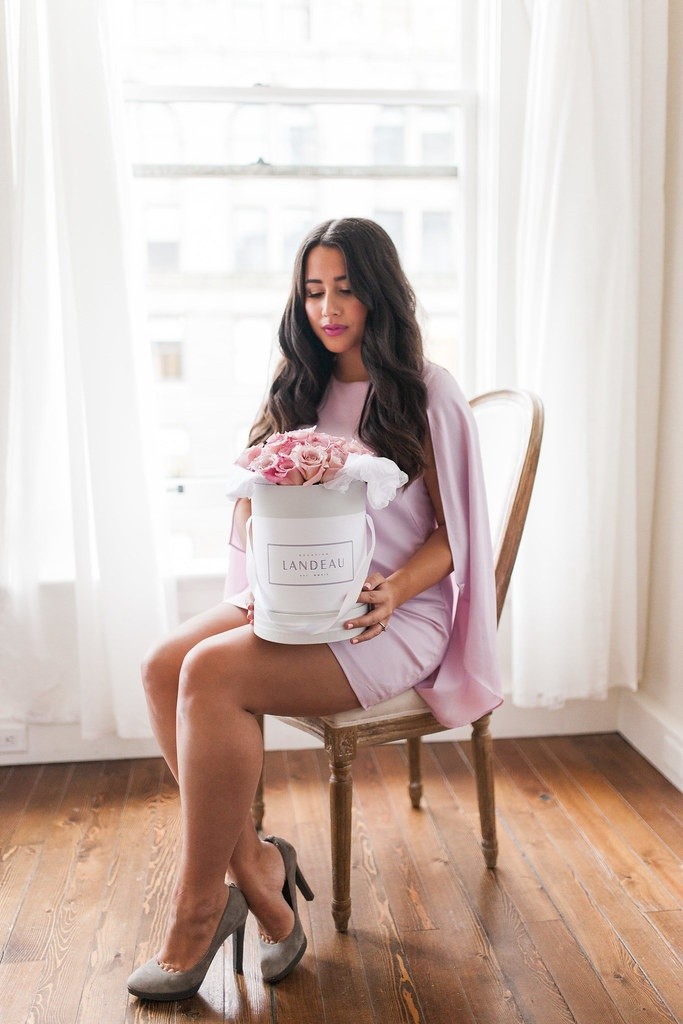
<box><xmin>127</xmin><ymin>834</ymin><xmax>315</xmax><ymax>1001</ymax></box>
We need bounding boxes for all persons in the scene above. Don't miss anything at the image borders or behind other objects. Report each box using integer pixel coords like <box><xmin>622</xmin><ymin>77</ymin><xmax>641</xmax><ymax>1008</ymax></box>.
<box><xmin>119</xmin><ymin>215</ymin><xmax>461</xmax><ymax>999</ymax></box>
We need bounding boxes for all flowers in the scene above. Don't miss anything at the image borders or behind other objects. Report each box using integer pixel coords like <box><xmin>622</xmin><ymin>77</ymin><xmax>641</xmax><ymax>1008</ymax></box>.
<box><xmin>236</xmin><ymin>423</ymin><xmax>374</xmax><ymax>488</ymax></box>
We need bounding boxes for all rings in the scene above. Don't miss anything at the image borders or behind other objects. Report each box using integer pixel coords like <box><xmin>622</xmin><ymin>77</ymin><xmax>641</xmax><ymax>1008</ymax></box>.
<box><xmin>379</xmin><ymin>621</ymin><xmax>389</xmax><ymax>632</ymax></box>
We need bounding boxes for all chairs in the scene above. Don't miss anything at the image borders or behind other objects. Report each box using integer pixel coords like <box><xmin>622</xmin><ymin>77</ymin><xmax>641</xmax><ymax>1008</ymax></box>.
<box><xmin>248</xmin><ymin>385</ymin><xmax>545</xmax><ymax>937</ymax></box>
